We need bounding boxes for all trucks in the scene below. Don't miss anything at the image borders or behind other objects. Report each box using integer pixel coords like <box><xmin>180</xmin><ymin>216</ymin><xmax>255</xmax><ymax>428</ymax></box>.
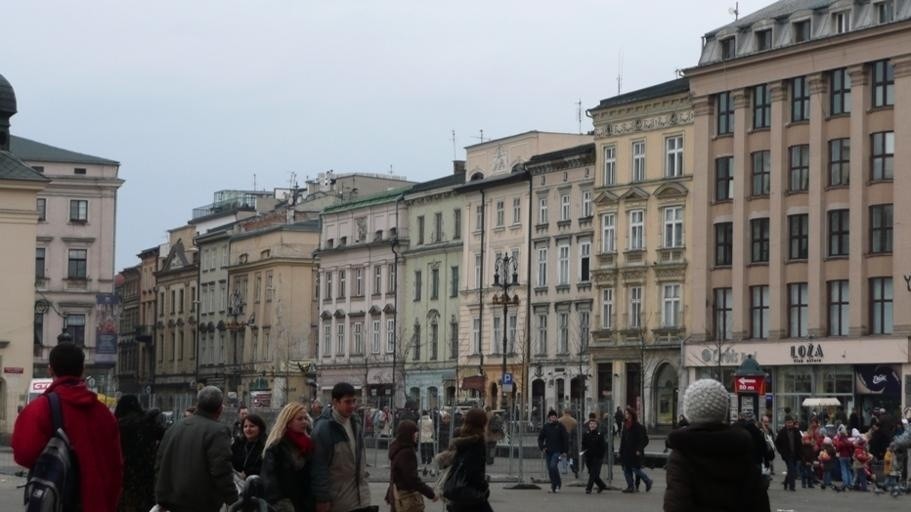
<box><xmin>14</xmin><ymin>377</ymin><xmax>53</xmax><ymax>414</ymax></box>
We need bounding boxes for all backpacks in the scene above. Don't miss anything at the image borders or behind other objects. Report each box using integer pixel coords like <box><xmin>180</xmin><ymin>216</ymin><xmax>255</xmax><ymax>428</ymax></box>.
<box><xmin>131</xmin><ymin>432</ymin><xmax>162</xmax><ymax>468</ymax></box>
<box><xmin>17</xmin><ymin>392</ymin><xmax>82</xmax><ymax>512</ymax></box>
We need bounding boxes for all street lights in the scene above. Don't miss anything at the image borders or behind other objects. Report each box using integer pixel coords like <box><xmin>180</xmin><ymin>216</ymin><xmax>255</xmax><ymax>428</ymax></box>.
<box><xmin>226</xmin><ymin>289</ymin><xmax>255</xmax><ymax>365</ymax></box>
<box><xmin>491</xmin><ymin>253</ymin><xmax>520</xmax><ymax>384</ymax></box>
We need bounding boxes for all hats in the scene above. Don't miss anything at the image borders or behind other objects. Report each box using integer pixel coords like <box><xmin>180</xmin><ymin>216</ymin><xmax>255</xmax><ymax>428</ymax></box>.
<box><xmin>115</xmin><ymin>395</ymin><xmax>144</xmax><ymax>419</ymax></box>
<box><xmin>683</xmin><ymin>379</ymin><xmax>728</xmax><ymax>424</ymax></box>
<box><xmin>397</xmin><ymin>420</ymin><xmax>419</xmax><ymax>434</ymax></box>
<box><xmin>548</xmin><ymin>410</ymin><xmax>557</xmax><ymax>417</ymax></box>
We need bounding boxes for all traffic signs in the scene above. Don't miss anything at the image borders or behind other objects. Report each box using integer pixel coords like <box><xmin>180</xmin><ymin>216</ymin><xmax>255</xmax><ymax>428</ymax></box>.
<box><xmin>732</xmin><ymin>374</ymin><xmax>765</xmax><ymax>396</ymax></box>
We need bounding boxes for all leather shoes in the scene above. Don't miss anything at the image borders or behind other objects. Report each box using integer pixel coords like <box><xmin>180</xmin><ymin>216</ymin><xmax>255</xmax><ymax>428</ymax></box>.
<box><xmin>549</xmin><ymin>480</ymin><xmax>652</xmax><ymax>493</ymax></box>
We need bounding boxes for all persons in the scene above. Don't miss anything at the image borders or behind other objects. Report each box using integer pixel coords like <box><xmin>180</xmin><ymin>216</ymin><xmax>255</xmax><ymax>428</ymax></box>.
<box><xmin>383</xmin><ymin>419</ymin><xmax>437</xmax><ymax>512</ymax></box>
<box><xmin>429</xmin><ymin>407</ymin><xmax>495</xmax><ymax>512</ymax></box>
<box><xmin>615</xmin><ymin>406</ymin><xmax>624</xmax><ymax>436</ymax></box>
<box><xmin>750</xmin><ymin>413</ymin><xmax>805</xmax><ymax>492</ymax></box>
<box><xmin>537</xmin><ymin>409</ymin><xmax>570</xmax><ymax>492</ymax></box>
<box><xmin>557</xmin><ymin>408</ymin><xmax>616</xmax><ymax>475</ymax></box>
<box><xmin>310</xmin><ymin>382</ymin><xmax>380</xmax><ymax>512</ymax></box>
<box><xmin>793</xmin><ymin>406</ymin><xmax>911</xmax><ymax>496</ymax></box>
<box><xmin>114</xmin><ymin>395</ymin><xmax>159</xmax><ymax>512</ymax></box>
<box><xmin>581</xmin><ymin>419</ymin><xmax>608</xmax><ymax>494</ymax></box>
<box><xmin>12</xmin><ymin>344</ymin><xmax>126</xmax><ymax>512</ymax></box>
<box><xmin>618</xmin><ymin>407</ymin><xmax>654</xmax><ymax>492</ymax></box>
<box><xmin>159</xmin><ymin>386</ymin><xmax>239</xmax><ymax>512</ymax></box>
<box><xmin>678</xmin><ymin>415</ymin><xmax>690</xmax><ymax>429</ymax></box>
<box><xmin>662</xmin><ymin>379</ymin><xmax>771</xmax><ymax>512</ymax></box>
<box><xmin>229</xmin><ymin>405</ymin><xmax>268</xmax><ymax>482</ymax></box>
<box><xmin>262</xmin><ymin>401</ymin><xmax>319</xmax><ymax>512</ymax></box>
<box><xmin>354</xmin><ymin>403</ymin><xmax>472</xmax><ymax>466</ymax></box>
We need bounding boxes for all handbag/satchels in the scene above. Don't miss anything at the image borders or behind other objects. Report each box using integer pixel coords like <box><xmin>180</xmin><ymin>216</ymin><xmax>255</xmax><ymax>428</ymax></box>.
<box><xmin>394</xmin><ymin>489</ymin><xmax>425</xmax><ymax>512</ymax></box>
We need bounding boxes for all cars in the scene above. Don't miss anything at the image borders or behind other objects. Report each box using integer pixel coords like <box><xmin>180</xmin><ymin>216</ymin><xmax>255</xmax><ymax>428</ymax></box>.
<box><xmin>454</xmin><ymin>405</ymin><xmax>473</xmax><ymax>416</ymax></box>
<box><xmin>161</xmin><ymin>409</ymin><xmax>190</xmax><ymax>425</ymax></box>
<box><xmin>369</xmin><ymin>408</ymin><xmax>388</xmax><ymax>428</ymax></box>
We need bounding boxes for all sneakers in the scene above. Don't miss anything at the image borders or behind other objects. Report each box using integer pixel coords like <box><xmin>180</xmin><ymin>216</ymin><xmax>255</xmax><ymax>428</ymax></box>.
<box><xmin>782</xmin><ymin>481</ymin><xmax>871</xmax><ymax>491</ymax></box>
